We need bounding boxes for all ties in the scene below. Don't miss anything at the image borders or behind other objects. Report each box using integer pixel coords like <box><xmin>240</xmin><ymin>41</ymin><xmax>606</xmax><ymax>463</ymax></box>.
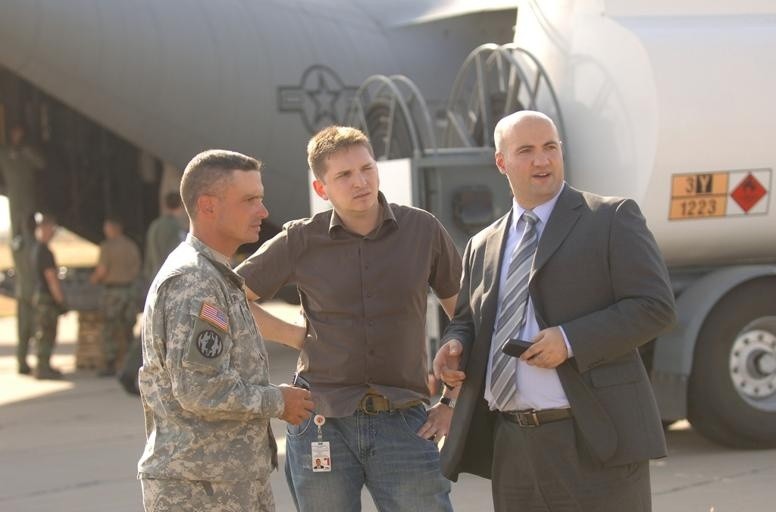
<box><xmin>488</xmin><ymin>209</ymin><xmax>540</xmax><ymax>412</ymax></box>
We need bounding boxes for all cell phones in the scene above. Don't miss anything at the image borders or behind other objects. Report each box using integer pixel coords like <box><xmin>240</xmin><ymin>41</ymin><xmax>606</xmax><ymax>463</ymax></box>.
<box><xmin>501</xmin><ymin>338</ymin><xmax>541</xmax><ymax>359</ymax></box>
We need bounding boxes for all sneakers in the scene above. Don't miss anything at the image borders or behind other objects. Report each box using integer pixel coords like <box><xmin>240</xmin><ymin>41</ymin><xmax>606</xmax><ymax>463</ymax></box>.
<box><xmin>16</xmin><ymin>362</ymin><xmax>118</xmax><ymax>381</ymax></box>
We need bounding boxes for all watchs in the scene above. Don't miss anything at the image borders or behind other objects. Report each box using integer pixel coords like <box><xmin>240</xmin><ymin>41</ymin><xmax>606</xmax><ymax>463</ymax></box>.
<box><xmin>439</xmin><ymin>396</ymin><xmax>458</xmax><ymax>409</ymax></box>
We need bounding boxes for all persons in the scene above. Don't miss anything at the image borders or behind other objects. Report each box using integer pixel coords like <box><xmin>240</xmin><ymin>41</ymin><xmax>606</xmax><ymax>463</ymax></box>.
<box><xmin>88</xmin><ymin>216</ymin><xmax>142</xmax><ymax>372</ymax></box>
<box><xmin>231</xmin><ymin>126</ymin><xmax>462</xmax><ymax>512</ymax></box>
<box><xmin>0</xmin><ymin>123</ymin><xmax>48</xmax><ymax>250</ymax></box>
<box><xmin>115</xmin><ymin>190</ymin><xmax>183</xmax><ymax>396</ymax></box>
<box><xmin>32</xmin><ymin>224</ymin><xmax>67</xmax><ymax>379</ymax></box>
<box><xmin>431</xmin><ymin>110</ymin><xmax>679</xmax><ymax>512</ymax></box>
<box><xmin>13</xmin><ymin>221</ymin><xmax>34</xmax><ymax>375</ymax></box>
<box><xmin>137</xmin><ymin>148</ymin><xmax>315</xmax><ymax>512</ymax></box>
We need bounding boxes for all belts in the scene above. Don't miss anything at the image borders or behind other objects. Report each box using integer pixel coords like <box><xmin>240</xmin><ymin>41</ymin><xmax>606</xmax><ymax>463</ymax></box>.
<box><xmin>355</xmin><ymin>394</ymin><xmax>422</xmax><ymax>416</ymax></box>
<box><xmin>499</xmin><ymin>409</ymin><xmax>573</xmax><ymax>425</ymax></box>
